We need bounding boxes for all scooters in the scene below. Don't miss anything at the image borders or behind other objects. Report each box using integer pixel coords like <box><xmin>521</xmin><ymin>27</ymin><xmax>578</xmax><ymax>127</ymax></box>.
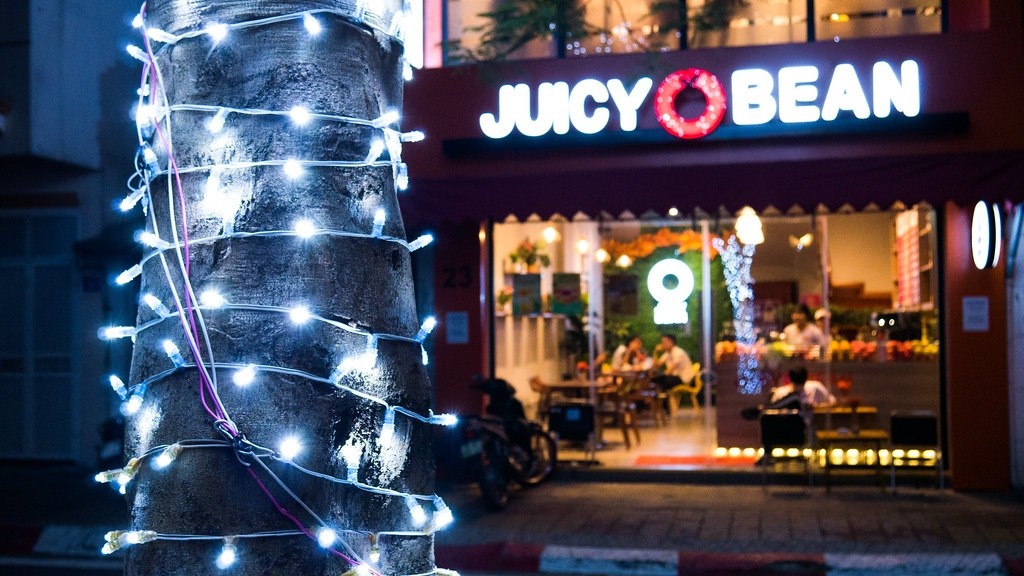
<box><xmin>453</xmin><ymin>373</ymin><xmax>560</xmax><ymax>512</ymax></box>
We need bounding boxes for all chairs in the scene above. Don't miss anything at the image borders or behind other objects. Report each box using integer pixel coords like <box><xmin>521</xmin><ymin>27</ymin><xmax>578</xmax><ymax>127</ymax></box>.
<box><xmin>597</xmin><ymin>362</ymin><xmax>703</xmax><ymax>448</ymax></box>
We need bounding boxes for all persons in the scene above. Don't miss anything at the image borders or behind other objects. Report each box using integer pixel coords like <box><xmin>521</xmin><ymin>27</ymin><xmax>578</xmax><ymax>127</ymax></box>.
<box><xmin>479</xmin><ymin>301</ymin><xmax>839</xmax><ymax>469</ymax></box>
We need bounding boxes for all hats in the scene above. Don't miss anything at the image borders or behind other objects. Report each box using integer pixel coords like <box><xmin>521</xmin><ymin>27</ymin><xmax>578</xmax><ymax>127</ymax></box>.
<box><xmin>814</xmin><ymin>308</ymin><xmax>831</xmax><ymax>319</ymax></box>
